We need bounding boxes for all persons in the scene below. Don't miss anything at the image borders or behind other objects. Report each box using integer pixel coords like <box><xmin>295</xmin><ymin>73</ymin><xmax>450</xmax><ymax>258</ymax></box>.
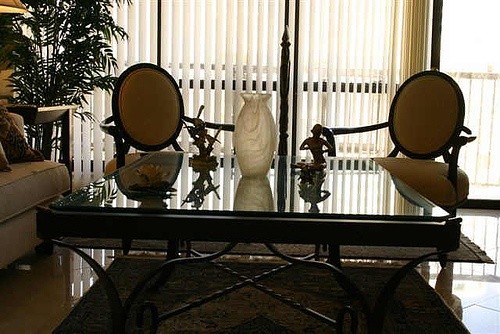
<box><xmin>300</xmin><ymin>124</ymin><xmax>333</xmax><ymax>186</ymax></box>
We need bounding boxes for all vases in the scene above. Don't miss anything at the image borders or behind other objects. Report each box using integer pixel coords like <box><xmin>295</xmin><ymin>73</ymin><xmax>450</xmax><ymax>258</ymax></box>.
<box><xmin>234</xmin><ymin>93</ymin><xmax>277</xmax><ymax>179</ymax></box>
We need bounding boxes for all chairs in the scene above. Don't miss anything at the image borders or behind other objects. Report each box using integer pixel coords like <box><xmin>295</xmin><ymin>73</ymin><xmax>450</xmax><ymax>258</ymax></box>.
<box><xmin>101</xmin><ymin>62</ymin><xmax>235</xmax><ymax>258</ymax></box>
<box><xmin>322</xmin><ymin>67</ymin><xmax>477</xmax><ymax>269</ymax></box>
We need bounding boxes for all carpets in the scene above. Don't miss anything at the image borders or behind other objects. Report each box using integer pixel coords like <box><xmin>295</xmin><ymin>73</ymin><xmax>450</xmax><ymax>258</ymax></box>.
<box><xmin>64</xmin><ymin>208</ymin><xmax>496</xmax><ymax>266</ymax></box>
<box><xmin>51</xmin><ymin>256</ymin><xmax>470</xmax><ymax>334</ymax></box>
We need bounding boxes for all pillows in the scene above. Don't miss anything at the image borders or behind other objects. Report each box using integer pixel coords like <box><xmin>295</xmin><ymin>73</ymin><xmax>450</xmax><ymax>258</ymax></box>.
<box><xmin>0</xmin><ymin>142</ymin><xmax>13</xmax><ymax>173</ymax></box>
<box><xmin>0</xmin><ymin>109</ymin><xmax>44</xmax><ymax>162</ymax></box>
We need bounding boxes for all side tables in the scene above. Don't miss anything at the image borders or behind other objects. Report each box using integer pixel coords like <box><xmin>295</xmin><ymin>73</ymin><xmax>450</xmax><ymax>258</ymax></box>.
<box><xmin>7</xmin><ymin>105</ymin><xmax>79</xmax><ymax>194</ymax></box>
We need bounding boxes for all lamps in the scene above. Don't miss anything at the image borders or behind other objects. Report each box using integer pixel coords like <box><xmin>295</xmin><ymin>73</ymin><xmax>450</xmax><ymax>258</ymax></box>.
<box><xmin>0</xmin><ymin>1</ymin><xmax>29</xmax><ymax>14</ymax></box>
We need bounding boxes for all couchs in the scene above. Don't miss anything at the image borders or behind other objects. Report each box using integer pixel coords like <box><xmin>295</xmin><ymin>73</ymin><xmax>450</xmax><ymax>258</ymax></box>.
<box><xmin>0</xmin><ymin>99</ymin><xmax>71</xmax><ymax>270</ymax></box>
